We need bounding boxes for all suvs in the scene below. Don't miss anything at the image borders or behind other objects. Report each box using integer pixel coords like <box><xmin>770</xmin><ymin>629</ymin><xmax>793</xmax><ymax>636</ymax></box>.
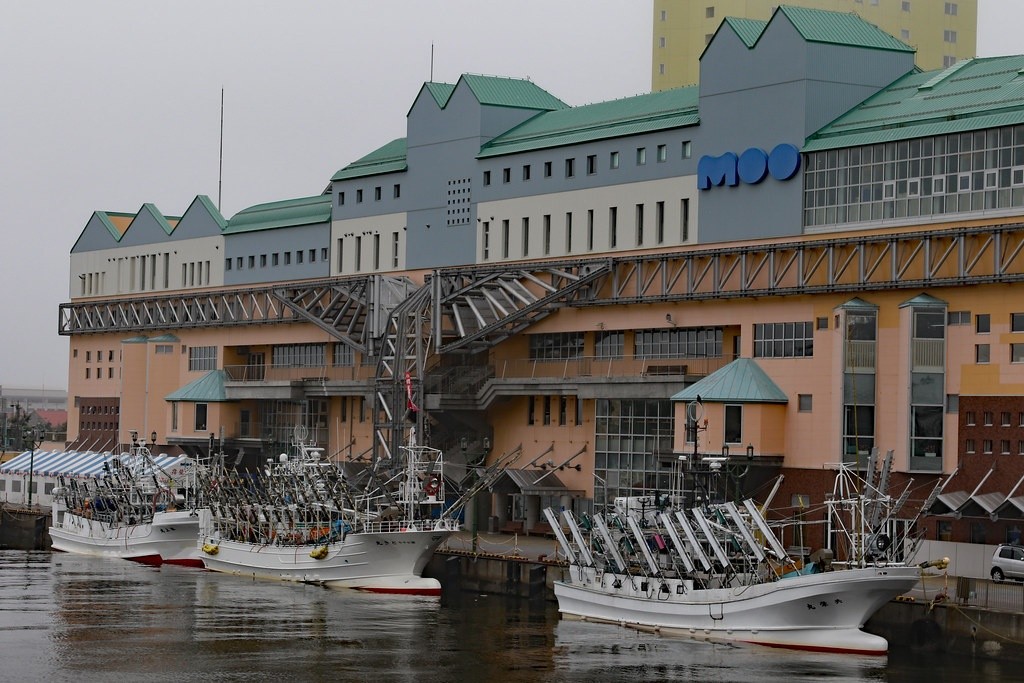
<box><xmin>989</xmin><ymin>543</ymin><xmax>1024</xmax><ymax>585</ymax></box>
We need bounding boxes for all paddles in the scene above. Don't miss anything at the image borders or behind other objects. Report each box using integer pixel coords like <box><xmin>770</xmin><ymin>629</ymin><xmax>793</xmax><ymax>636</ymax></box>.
<box><xmin>60</xmin><ymin>466</ymin><xmax>153</xmax><ymax>531</ymax></box>
<box><xmin>364</xmin><ymin>460</ymin><xmax>436</xmax><ymax>521</ymax></box>
<box><xmin>848</xmin><ymin>445</ymin><xmax>943</xmax><ymax>564</ymax></box>
<box><xmin>195</xmin><ymin>464</ymin><xmax>358</xmax><ymax>547</ymax></box>
<box><xmin>541</xmin><ymin>495</ymin><xmax>801</xmax><ymax>596</ymax></box>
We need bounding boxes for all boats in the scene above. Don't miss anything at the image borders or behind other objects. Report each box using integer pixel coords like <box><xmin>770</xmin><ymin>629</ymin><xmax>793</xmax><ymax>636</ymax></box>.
<box><xmin>540</xmin><ymin>401</ymin><xmax>953</xmax><ymax>659</ymax></box>
<box><xmin>45</xmin><ymin>436</ymin><xmax>205</xmax><ymax>571</ymax></box>
<box><xmin>195</xmin><ymin>417</ymin><xmax>475</xmax><ymax>597</ymax></box>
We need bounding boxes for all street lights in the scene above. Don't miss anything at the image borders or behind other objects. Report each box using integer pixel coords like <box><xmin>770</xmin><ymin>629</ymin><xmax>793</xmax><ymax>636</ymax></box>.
<box><xmin>721</xmin><ymin>443</ymin><xmax>755</xmax><ymax>508</ymax></box>
<box><xmin>460</xmin><ymin>437</ymin><xmax>492</xmax><ymax>553</ymax></box>
<box><xmin>21</xmin><ymin>427</ymin><xmax>45</xmax><ymax>511</ymax></box>
<box><xmin>131</xmin><ymin>429</ymin><xmax>158</xmax><ymax>490</ymax></box>
